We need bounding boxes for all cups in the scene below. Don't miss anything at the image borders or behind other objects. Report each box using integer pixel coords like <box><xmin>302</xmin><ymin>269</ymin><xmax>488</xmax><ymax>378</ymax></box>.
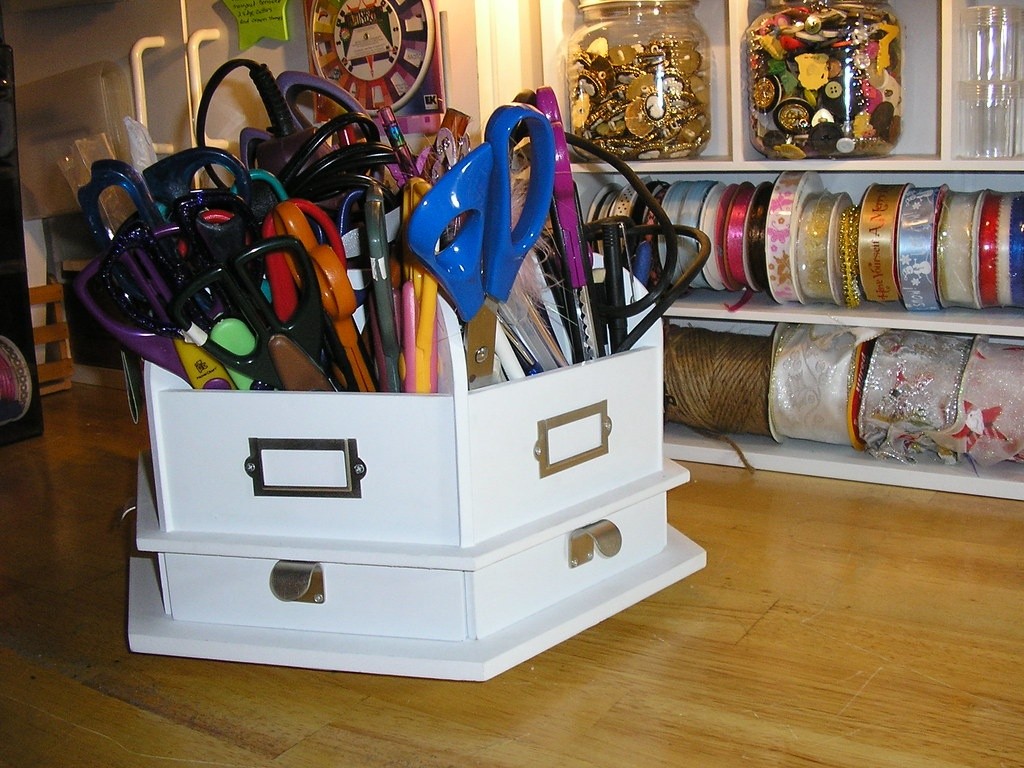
<box><xmin>963</xmin><ymin>4</ymin><xmax>1017</xmax><ymax>80</ymax></box>
<box><xmin>957</xmin><ymin>82</ymin><xmax>1015</xmax><ymax>160</ymax></box>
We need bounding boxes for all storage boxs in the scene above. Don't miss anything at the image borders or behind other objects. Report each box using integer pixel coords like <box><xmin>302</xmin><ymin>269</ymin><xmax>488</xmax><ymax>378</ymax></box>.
<box><xmin>120</xmin><ymin>252</ymin><xmax>708</xmax><ymax>682</ymax></box>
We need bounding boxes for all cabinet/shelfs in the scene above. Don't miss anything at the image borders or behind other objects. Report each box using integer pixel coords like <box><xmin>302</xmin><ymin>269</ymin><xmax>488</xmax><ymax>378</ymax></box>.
<box><xmin>532</xmin><ymin>0</ymin><xmax>1024</xmax><ymax>500</ymax></box>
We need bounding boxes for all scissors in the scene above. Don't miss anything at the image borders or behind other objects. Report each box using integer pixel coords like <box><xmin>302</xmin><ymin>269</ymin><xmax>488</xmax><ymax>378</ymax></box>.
<box><xmin>79</xmin><ymin>72</ymin><xmax>596</xmax><ymax>395</ymax></box>
